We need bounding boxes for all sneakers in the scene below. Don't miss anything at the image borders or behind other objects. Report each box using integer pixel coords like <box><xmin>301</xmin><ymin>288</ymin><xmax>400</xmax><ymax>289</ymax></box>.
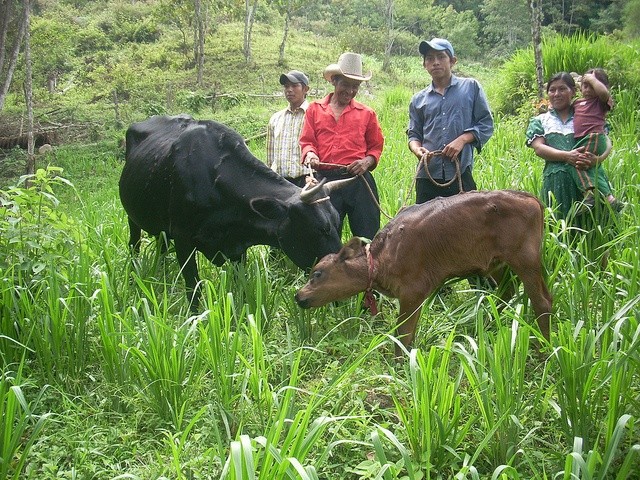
<box><xmin>610</xmin><ymin>198</ymin><xmax>625</xmax><ymax>214</ymax></box>
<box><xmin>576</xmin><ymin>196</ymin><xmax>596</xmax><ymax>216</ymax></box>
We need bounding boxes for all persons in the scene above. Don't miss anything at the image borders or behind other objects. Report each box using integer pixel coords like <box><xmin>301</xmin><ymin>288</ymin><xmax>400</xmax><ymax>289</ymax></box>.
<box><xmin>547</xmin><ymin>68</ymin><xmax>624</xmax><ymax>217</ymax></box>
<box><xmin>405</xmin><ymin>37</ymin><xmax>501</xmax><ymax>295</ymax></box>
<box><xmin>266</xmin><ymin>70</ymin><xmax>312</xmax><ymax>268</ymax></box>
<box><xmin>297</xmin><ymin>52</ymin><xmax>383</xmax><ymax>311</ymax></box>
<box><xmin>526</xmin><ymin>71</ymin><xmax>612</xmax><ymax>236</ymax></box>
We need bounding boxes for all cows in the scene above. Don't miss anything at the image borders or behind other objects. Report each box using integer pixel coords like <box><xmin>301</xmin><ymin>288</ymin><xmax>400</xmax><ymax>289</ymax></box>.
<box><xmin>118</xmin><ymin>112</ymin><xmax>357</xmax><ymax>319</ymax></box>
<box><xmin>295</xmin><ymin>190</ymin><xmax>554</xmax><ymax>369</ymax></box>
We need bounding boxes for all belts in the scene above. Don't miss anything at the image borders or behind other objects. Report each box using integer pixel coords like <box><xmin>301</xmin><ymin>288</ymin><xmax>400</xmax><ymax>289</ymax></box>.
<box><xmin>319</xmin><ymin>167</ymin><xmax>349</xmax><ymax>177</ymax></box>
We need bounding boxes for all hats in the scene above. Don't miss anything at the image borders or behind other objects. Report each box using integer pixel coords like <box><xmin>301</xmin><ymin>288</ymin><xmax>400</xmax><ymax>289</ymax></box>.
<box><xmin>419</xmin><ymin>38</ymin><xmax>454</xmax><ymax>57</ymax></box>
<box><xmin>280</xmin><ymin>70</ymin><xmax>309</xmax><ymax>86</ymax></box>
<box><xmin>323</xmin><ymin>52</ymin><xmax>372</xmax><ymax>84</ymax></box>
